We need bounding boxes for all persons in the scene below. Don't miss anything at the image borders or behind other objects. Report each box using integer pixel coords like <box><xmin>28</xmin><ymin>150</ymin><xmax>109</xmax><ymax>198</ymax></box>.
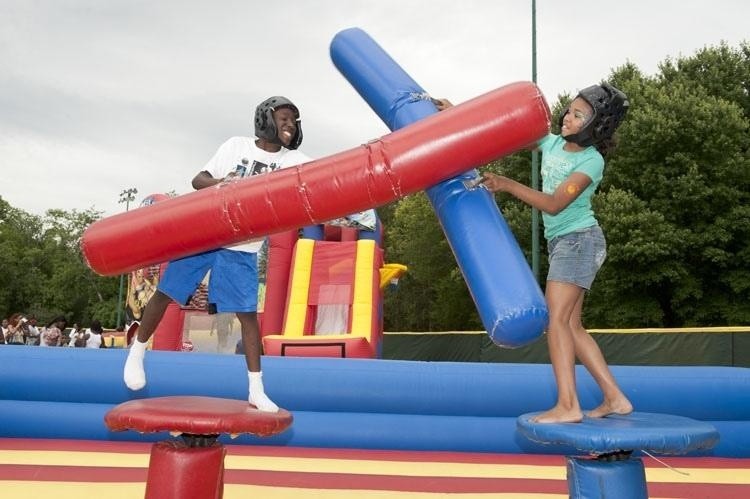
<box><xmin>0</xmin><ymin>312</ymin><xmax>107</xmax><ymax>349</ymax></box>
<box><xmin>431</xmin><ymin>80</ymin><xmax>635</xmax><ymax>426</ymax></box>
<box><xmin>122</xmin><ymin>94</ymin><xmax>383</xmax><ymax>415</ymax></box>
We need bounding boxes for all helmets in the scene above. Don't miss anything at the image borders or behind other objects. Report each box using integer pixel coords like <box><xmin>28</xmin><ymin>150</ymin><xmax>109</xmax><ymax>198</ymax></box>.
<box><xmin>560</xmin><ymin>83</ymin><xmax>629</xmax><ymax>146</ymax></box>
<box><xmin>255</xmin><ymin>96</ymin><xmax>302</xmax><ymax>151</ymax></box>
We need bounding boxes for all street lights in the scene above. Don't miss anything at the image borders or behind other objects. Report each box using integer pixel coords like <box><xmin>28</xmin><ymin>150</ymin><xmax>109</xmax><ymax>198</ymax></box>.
<box><xmin>117</xmin><ymin>188</ymin><xmax>138</xmax><ymax>329</ymax></box>
